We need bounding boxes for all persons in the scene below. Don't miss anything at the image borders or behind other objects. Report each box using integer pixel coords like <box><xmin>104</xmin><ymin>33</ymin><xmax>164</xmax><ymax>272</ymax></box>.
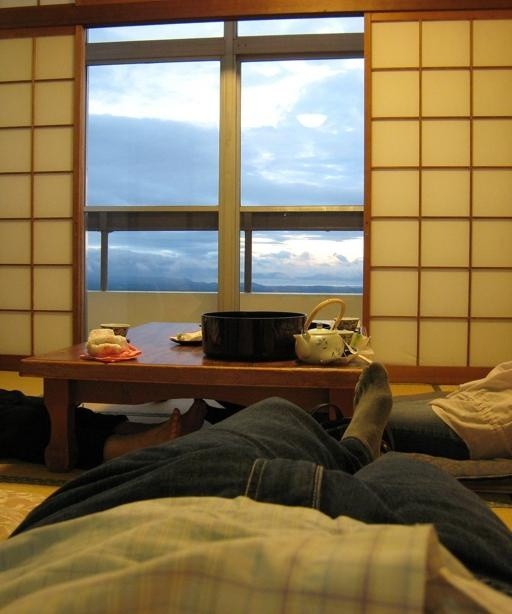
<box><xmin>0</xmin><ymin>363</ymin><xmax>511</xmax><ymax>613</ymax></box>
<box><xmin>0</xmin><ymin>388</ymin><xmax>208</xmax><ymax>471</ymax></box>
<box><xmin>194</xmin><ymin>360</ymin><xmax>512</xmax><ymax>461</ymax></box>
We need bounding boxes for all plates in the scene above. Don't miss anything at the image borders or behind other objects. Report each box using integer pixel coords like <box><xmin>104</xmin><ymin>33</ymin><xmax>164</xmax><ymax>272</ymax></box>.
<box><xmin>171</xmin><ymin>337</ymin><xmax>202</xmax><ymax>347</ymax></box>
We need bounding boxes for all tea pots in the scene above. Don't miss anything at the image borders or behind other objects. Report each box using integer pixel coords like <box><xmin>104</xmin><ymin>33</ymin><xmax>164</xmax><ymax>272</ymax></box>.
<box><xmin>294</xmin><ymin>298</ymin><xmax>346</xmax><ymax>365</ymax></box>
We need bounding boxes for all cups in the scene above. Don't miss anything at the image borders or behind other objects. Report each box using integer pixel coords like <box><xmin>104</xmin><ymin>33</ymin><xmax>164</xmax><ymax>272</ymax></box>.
<box><xmin>333</xmin><ymin>316</ymin><xmax>371</xmax><ymax>350</ymax></box>
<box><xmin>101</xmin><ymin>323</ymin><xmax>130</xmax><ymax>339</ymax></box>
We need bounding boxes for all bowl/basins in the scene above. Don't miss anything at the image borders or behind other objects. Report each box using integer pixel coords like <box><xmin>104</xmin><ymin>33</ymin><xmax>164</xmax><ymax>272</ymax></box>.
<box><xmin>201</xmin><ymin>311</ymin><xmax>308</xmax><ymax>362</ymax></box>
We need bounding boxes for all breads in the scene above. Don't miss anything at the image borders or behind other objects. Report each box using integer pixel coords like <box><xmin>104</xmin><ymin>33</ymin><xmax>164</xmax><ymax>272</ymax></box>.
<box><xmin>177</xmin><ymin>331</ymin><xmax>203</xmax><ymax>340</ymax></box>
<box><xmin>88</xmin><ymin>328</ymin><xmax>127</xmax><ymax>355</ymax></box>
